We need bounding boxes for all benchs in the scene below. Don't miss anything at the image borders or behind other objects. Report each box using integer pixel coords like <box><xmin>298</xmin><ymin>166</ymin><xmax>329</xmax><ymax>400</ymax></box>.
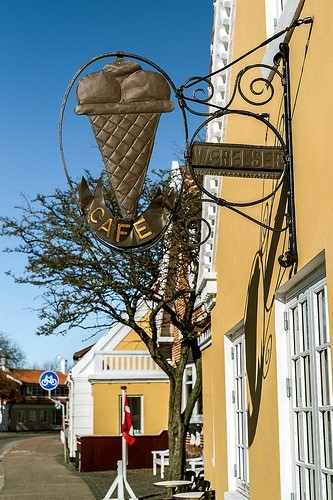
<box><xmin>151</xmin><ymin>426</ymin><xmax>205</xmax><ymax>481</ymax></box>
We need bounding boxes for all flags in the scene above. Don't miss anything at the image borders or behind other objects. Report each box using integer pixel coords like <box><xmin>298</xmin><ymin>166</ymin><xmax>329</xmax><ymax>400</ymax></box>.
<box><xmin>121</xmin><ymin>394</ymin><xmax>136</xmax><ymax>446</ymax></box>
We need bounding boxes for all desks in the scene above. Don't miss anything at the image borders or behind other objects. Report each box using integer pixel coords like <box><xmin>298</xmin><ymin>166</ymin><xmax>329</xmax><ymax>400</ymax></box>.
<box><xmin>153</xmin><ymin>481</ymin><xmax>205</xmax><ymax>500</ymax></box>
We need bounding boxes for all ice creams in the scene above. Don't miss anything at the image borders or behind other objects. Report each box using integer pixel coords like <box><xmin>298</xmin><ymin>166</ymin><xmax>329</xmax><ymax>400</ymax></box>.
<box><xmin>74</xmin><ymin>58</ymin><xmax>175</xmax><ymax>221</ymax></box>
